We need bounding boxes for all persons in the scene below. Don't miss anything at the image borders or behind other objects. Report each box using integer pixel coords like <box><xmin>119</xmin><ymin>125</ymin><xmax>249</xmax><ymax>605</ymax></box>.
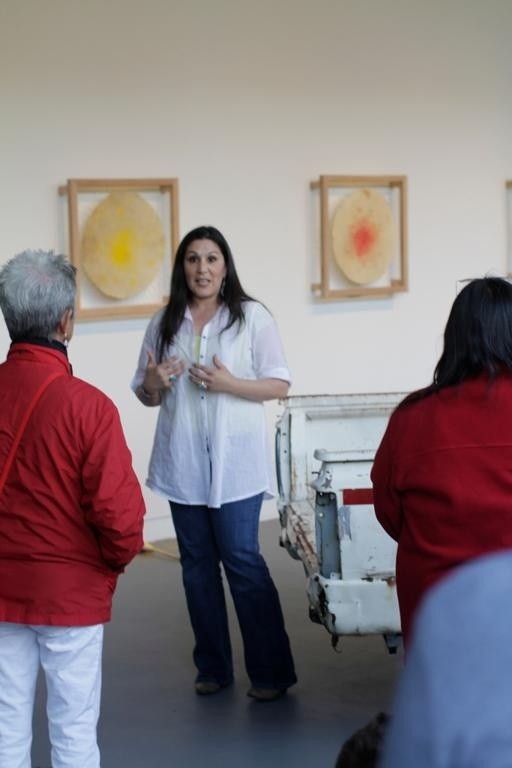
<box><xmin>130</xmin><ymin>225</ymin><xmax>298</xmax><ymax>700</ymax></box>
<box><xmin>1</xmin><ymin>247</ymin><xmax>146</xmax><ymax>768</ymax></box>
<box><xmin>379</xmin><ymin>547</ymin><xmax>511</xmax><ymax>768</ymax></box>
<box><xmin>335</xmin><ymin>711</ymin><xmax>387</xmax><ymax>767</ymax></box>
<box><xmin>370</xmin><ymin>276</ymin><xmax>512</xmax><ymax>650</ymax></box>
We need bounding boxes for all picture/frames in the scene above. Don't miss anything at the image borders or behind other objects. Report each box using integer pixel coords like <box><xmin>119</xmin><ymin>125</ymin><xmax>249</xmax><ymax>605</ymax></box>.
<box><xmin>59</xmin><ymin>177</ymin><xmax>182</xmax><ymax>322</ymax></box>
<box><xmin>310</xmin><ymin>172</ymin><xmax>409</xmax><ymax>298</ymax></box>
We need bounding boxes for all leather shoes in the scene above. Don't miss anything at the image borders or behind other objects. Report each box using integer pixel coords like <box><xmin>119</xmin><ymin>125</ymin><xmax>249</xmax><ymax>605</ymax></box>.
<box><xmin>196</xmin><ymin>681</ymin><xmax>220</xmax><ymax>694</ymax></box>
<box><xmin>247</xmin><ymin>686</ymin><xmax>281</xmax><ymax>700</ymax></box>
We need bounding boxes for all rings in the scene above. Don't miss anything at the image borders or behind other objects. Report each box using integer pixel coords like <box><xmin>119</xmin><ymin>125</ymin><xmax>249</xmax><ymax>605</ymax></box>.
<box><xmin>198</xmin><ymin>381</ymin><xmax>207</xmax><ymax>390</ymax></box>
<box><xmin>169</xmin><ymin>374</ymin><xmax>176</xmax><ymax>381</ymax></box>
